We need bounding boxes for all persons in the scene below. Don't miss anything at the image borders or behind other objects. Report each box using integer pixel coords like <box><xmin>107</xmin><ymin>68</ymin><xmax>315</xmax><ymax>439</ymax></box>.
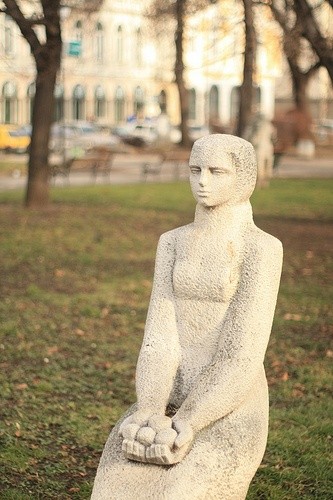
<box><xmin>88</xmin><ymin>133</ymin><xmax>285</xmax><ymax>500</ymax></box>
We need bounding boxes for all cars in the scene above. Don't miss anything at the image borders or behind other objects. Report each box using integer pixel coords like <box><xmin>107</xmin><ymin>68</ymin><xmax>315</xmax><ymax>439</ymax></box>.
<box><xmin>310</xmin><ymin>118</ymin><xmax>333</xmax><ymax>146</ymax></box>
<box><xmin>50</xmin><ymin>110</ymin><xmax>227</xmax><ymax>154</ymax></box>
<box><xmin>0</xmin><ymin>123</ymin><xmax>31</xmax><ymax>153</ymax></box>
